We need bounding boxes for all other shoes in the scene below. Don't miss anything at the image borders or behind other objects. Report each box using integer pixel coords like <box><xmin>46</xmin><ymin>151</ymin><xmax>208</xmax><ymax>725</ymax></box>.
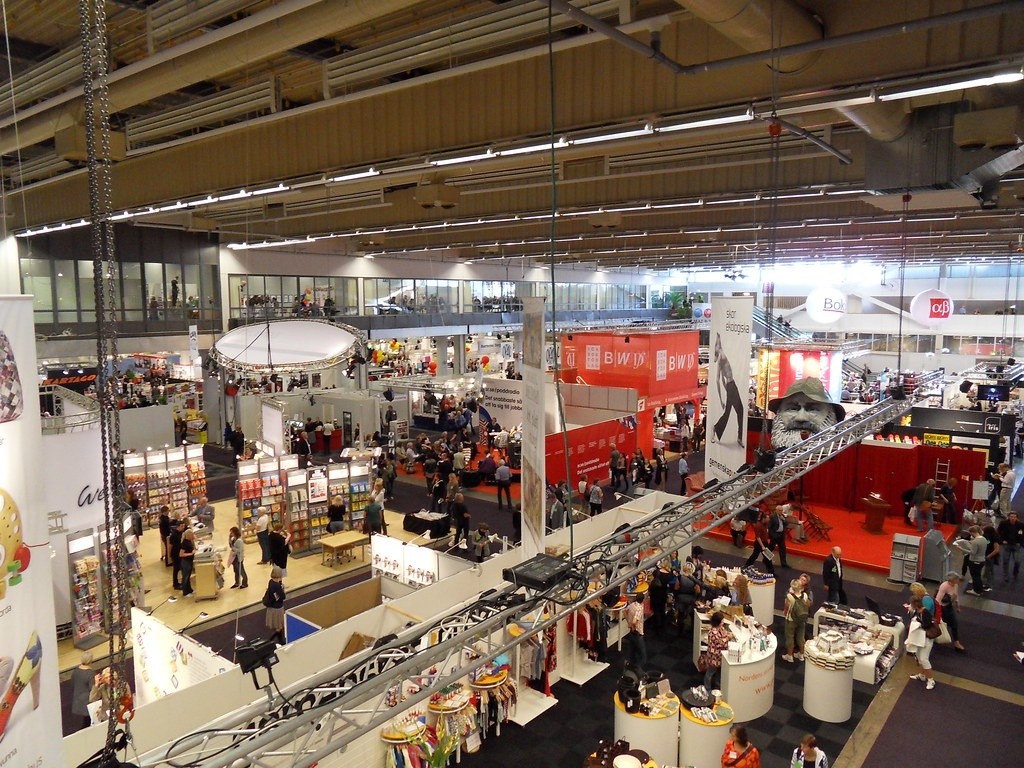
<box><xmin>239</xmin><ymin>584</ymin><xmax>249</xmax><ymax>589</ymax></box>
<box><xmin>954</xmin><ymin>641</ymin><xmax>964</xmax><ymax>649</ymax></box>
<box><xmin>966</xmin><ymin>589</ymin><xmax>980</xmax><ymax>596</ymax></box>
<box><xmin>983</xmin><ymin>585</ymin><xmax>993</xmax><ymax>591</ymax></box>
<box><xmin>231</xmin><ymin>585</ymin><xmax>238</xmax><ymax>588</ymax></box>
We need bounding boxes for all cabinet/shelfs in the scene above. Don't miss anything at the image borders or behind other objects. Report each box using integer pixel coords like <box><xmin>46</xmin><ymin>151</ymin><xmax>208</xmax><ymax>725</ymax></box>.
<box><xmin>693</xmin><ymin>608</ymin><xmax>778</xmax><ymax>723</ymax></box>
<box><xmin>389</xmin><ymin>419</ymin><xmax>410</xmax><ymax>442</ymax></box>
<box><xmin>65</xmin><ymin>446</ymin><xmax>372</xmax><ymax>651</ymax></box>
<box><xmin>889</xmin><ymin>534</ymin><xmax>921</xmax><ymax>584</ymax></box>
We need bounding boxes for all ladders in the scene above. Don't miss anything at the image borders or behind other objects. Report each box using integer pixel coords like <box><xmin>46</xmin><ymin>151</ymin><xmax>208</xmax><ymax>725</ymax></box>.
<box><xmin>932</xmin><ymin>458</ymin><xmax>950</xmax><ymax>501</ymax></box>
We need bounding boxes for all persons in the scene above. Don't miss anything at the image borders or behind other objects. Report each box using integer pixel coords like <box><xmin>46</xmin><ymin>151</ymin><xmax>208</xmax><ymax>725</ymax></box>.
<box><xmin>617</xmin><ymin>502</ymin><xmax>848</xmax><ymax>693</ymax></box>
<box><xmin>363</xmin><ymin>392</ymin><xmax>521</xmax><ymax>563</ymax></box>
<box><xmin>38</xmin><ymin>358</ymin><xmax>170</xmax><ymax>417</ymax></box>
<box><xmin>124</xmin><ymin>487</ymin><xmax>249</xmax><ymax>597</ymax></box>
<box><xmin>606</xmin><ymin>401</ymin><xmax>706</xmax><ymax>493</ymax></box>
<box><xmin>958</xmin><ymin>304</ymin><xmax>1016</xmax><ymax>315</ymax></box>
<box><xmin>327</xmin><ymin>494</ymin><xmax>346</xmax><ymax>533</ymax></box>
<box><xmin>366</xmin><ymin>331</ymin><xmax>522</xmax><ymax>381</ymax></box>
<box><xmin>145</xmin><ymin>275</ymin><xmax>524</xmax><ymax>321</ymax></box>
<box><xmin>546</xmin><ymin>474</ymin><xmax>603</xmax><ymax>536</ymax></box>
<box><xmin>255</xmin><ymin>506</ymin><xmax>292</xmax><ymax>646</ymax></box>
<box><xmin>767</xmin><ymin>376</ymin><xmax>845</xmax><ymax>455</ymax></box>
<box><xmin>224</xmin><ymin>368</ymin><xmax>362</xmax><ymax>468</ymax></box>
<box><xmin>775</xmin><ymin>313</ymin><xmax>792</xmax><ymax>335</ymax></box>
<box><xmin>840</xmin><ymin>363</ymin><xmax>1024</xmax><ymax>417</ymax></box>
<box><xmin>900</xmin><ymin>462</ymin><xmax>1024</xmax><ymax>689</ymax></box>
<box><xmin>711</xmin><ymin>333</ymin><xmax>744</xmax><ymax>448</ymax></box>
<box><xmin>721</xmin><ymin>724</ymin><xmax>829</xmax><ymax>768</ymax></box>
<box><xmin>174</xmin><ymin>416</ymin><xmax>188</xmax><ymax>447</ymax></box>
<box><xmin>68</xmin><ymin>651</ymin><xmax>133</xmax><ymax>723</ymax></box>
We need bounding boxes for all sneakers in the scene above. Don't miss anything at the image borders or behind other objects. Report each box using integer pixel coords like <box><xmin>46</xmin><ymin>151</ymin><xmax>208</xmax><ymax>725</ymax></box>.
<box><xmin>926</xmin><ymin>678</ymin><xmax>935</xmax><ymax>690</ymax></box>
<box><xmin>910</xmin><ymin>672</ymin><xmax>926</xmax><ymax>681</ymax></box>
<box><xmin>782</xmin><ymin>654</ymin><xmax>794</xmax><ymax>662</ymax></box>
<box><xmin>793</xmin><ymin>652</ymin><xmax>804</xmax><ymax>661</ymax></box>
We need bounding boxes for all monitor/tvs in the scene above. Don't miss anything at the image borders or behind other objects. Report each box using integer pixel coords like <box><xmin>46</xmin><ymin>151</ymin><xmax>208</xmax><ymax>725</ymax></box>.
<box><xmin>289</xmin><ymin>420</ymin><xmax>305</xmax><ymax>431</ymax></box>
<box><xmin>199</xmin><ymin>423</ymin><xmax>206</xmax><ymax>428</ymax></box>
<box><xmin>977</xmin><ymin>385</ymin><xmax>1009</xmax><ymax>401</ymax></box>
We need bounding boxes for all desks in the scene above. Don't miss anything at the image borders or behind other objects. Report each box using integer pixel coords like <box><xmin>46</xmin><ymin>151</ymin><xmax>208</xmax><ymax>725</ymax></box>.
<box><xmin>804</xmin><ymin>640</ymin><xmax>853</xmax><ymax>723</ymax></box>
<box><xmin>192</xmin><ymin>556</ymin><xmax>219</xmax><ymax>602</ymax></box>
<box><xmin>710</xmin><ymin>568</ymin><xmax>776</xmax><ymax>626</ymax></box>
<box><xmin>317</xmin><ymin>531</ymin><xmax>369</xmax><ymax>571</ymax></box>
<box><xmin>813</xmin><ymin>606</ymin><xmax>907</xmax><ymax>685</ymax></box>
<box><xmin>679</xmin><ymin>701</ymin><xmax>734</xmax><ymax>768</ymax></box>
<box><xmin>614</xmin><ymin>690</ymin><xmax>681</xmax><ymax>768</ymax></box>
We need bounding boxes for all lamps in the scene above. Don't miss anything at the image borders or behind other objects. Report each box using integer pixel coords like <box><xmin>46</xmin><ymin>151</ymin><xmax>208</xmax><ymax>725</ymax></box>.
<box><xmin>488</xmin><ymin>533</ymin><xmax>517</xmax><ymax>549</ymax></box>
<box><xmin>145</xmin><ymin>595</ymin><xmax>177</xmax><ymax>616</ymax></box>
<box><xmin>890</xmin><ymin>386</ymin><xmax>907</xmax><ymax>401</ymax></box>
<box><xmin>215</xmin><ymin>632</ymin><xmax>245</xmax><ymax>655</ymax></box>
<box><xmin>423</xmin><ymin>391</ymin><xmax>438</xmax><ymax>406</ymax></box>
<box><xmin>571</xmin><ymin>508</ymin><xmax>592</xmax><ymax>517</ymax></box>
<box><xmin>234</xmin><ymin>636</ymin><xmax>279</xmax><ymax>698</ymax></box>
<box><xmin>576</xmin><ymin>375</ymin><xmax>588</xmax><ymax>387</ymax></box>
<box><xmin>382</xmin><ymin>386</ymin><xmax>395</xmax><ymax>402</ymax></box>
<box><xmin>1006</xmin><ymin>358</ymin><xmax>1015</xmax><ymax>365</ymax></box>
<box><xmin>309</xmin><ymin>395</ymin><xmax>317</xmax><ymax>407</ymax></box>
<box><xmin>417</xmin><ymin>339</ymin><xmax>421</xmax><ymax>344</ymax></box>
<box><xmin>161</xmin><ymin>442</ymin><xmax>169</xmax><ymax>449</ymax></box>
<box><xmin>730</xmin><ymin>271</ymin><xmax>748</xmax><ymax>282</ymax></box>
<box><xmin>625</xmin><ymin>335</ymin><xmax>630</xmax><ymax>343</ymax></box>
<box><xmin>496</xmin><ymin>333</ymin><xmax>501</xmax><ymax>340</ymax></box>
<box><xmin>177</xmin><ymin>611</ymin><xmax>209</xmax><ymax>632</ymax></box>
<box><xmin>201</xmin><ymin>346</ymin><xmax>375</xmax><ymax>392</ymax></box>
<box><xmin>468</xmin><ymin>335</ymin><xmax>472</xmax><ymax>340</ymax></box>
<box><xmin>443</xmin><ymin>538</ymin><xmax>468</xmax><ymax>555</ymax></box>
<box><xmin>959</xmin><ymin>380</ymin><xmax>974</xmax><ymax>395</ymax></box>
<box><xmin>732</xmin><ymin>505</ymin><xmax>766</xmax><ymax>524</ymax></box>
<box><xmin>506</xmin><ymin>332</ymin><xmax>510</xmax><ymax>339</ymax></box>
<box><xmin>568</xmin><ymin>333</ymin><xmax>573</xmax><ymax>340</ymax></box>
<box><xmin>236</xmin><ymin>454</ymin><xmax>246</xmax><ymax>461</ymax></box>
<box><xmin>996</xmin><ymin>366</ymin><xmax>1005</xmax><ymax>372</ymax></box>
<box><xmin>14</xmin><ymin>60</ymin><xmax>1024</xmax><ymax>268</ymax></box>
<box><xmin>329</xmin><ymin>458</ymin><xmax>336</xmax><ymax>463</ymax></box>
<box><xmin>351</xmin><ymin>455</ymin><xmax>360</xmax><ymax>461</ymax></box>
<box><xmin>615</xmin><ymin>493</ymin><xmax>636</xmax><ymax>501</ymax></box>
<box><xmin>182</xmin><ymin>440</ymin><xmax>194</xmax><ymax>446</ymax></box>
<box><xmin>433</xmin><ymin>338</ymin><xmax>436</xmax><ymax>344</ymax></box>
<box><xmin>407</xmin><ymin>530</ymin><xmax>431</xmax><ymax>544</ymax></box>
<box><xmin>307</xmin><ymin>461</ymin><xmax>314</xmax><ymax>467</ymax></box>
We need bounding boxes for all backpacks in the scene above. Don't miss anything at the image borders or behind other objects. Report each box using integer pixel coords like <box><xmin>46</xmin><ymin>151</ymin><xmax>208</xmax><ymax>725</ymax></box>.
<box><xmin>790</xmin><ymin>591</ymin><xmax>809</xmax><ymax>625</ymax></box>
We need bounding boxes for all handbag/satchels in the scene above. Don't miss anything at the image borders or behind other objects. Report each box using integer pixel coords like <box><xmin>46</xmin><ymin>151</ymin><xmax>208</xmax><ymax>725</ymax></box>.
<box><xmin>916</xmin><ymin>610</ymin><xmax>942</xmax><ymax>638</ymax></box>
<box><xmin>934</xmin><ymin>591</ymin><xmax>951</xmax><ymax>603</ymax></box>
<box><xmin>923</xmin><ymin>594</ymin><xmax>942</xmax><ymax>624</ymax></box>
<box><xmin>362</xmin><ymin>519</ymin><xmax>367</xmax><ymax>534</ymax></box>
<box><xmin>933</xmin><ymin>621</ymin><xmax>952</xmax><ymax>643</ymax></box>
<box><xmin>920</xmin><ymin>501</ymin><xmax>932</xmax><ymax>515</ymax></box>
<box><xmin>584</xmin><ymin>492</ymin><xmax>590</xmax><ymax>502</ymax></box>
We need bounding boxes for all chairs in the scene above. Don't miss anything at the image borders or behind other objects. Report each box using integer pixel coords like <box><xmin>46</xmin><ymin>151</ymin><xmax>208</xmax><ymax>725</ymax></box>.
<box><xmin>322</xmin><ymin>531</ymin><xmax>357</xmax><ymax>567</ymax></box>
<box><xmin>684</xmin><ymin>472</ymin><xmax>705</xmax><ymax>503</ymax></box>
<box><xmin>785</xmin><ymin>504</ymin><xmax>832</xmax><ymax>545</ymax></box>
